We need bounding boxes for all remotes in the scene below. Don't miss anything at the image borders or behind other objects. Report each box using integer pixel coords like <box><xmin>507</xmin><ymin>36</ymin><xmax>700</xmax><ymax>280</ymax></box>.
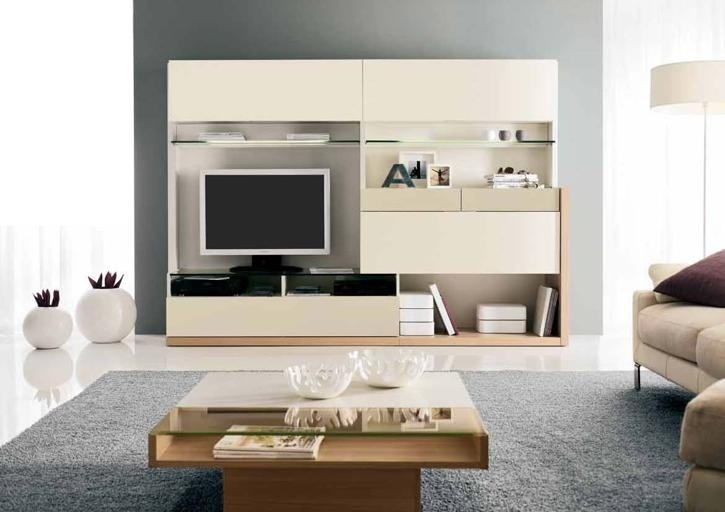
<box><xmin>309</xmin><ymin>265</ymin><xmax>353</xmax><ymax>273</ymax></box>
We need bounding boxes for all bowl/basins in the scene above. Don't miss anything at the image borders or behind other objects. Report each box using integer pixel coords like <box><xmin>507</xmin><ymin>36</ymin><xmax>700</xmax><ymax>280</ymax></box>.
<box><xmin>346</xmin><ymin>347</ymin><xmax>431</xmax><ymax>389</ymax></box>
<box><xmin>282</xmin><ymin>359</ymin><xmax>362</xmax><ymax>400</ymax></box>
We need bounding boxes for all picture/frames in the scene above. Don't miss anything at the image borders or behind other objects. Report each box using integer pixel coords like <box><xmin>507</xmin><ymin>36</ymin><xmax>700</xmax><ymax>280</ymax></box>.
<box><xmin>426</xmin><ymin>164</ymin><xmax>452</xmax><ymax>189</ymax></box>
<box><xmin>399</xmin><ymin>151</ymin><xmax>436</xmax><ymax>182</ymax></box>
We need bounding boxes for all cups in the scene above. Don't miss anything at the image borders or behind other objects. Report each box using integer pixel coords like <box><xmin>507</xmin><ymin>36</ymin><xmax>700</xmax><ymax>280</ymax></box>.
<box><xmin>515</xmin><ymin>129</ymin><xmax>529</xmax><ymax>141</ymax></box>
<box><xmin>498</xmin><ymin>130</ymin><xmax>512</xmax><ymax>141</ymax></box>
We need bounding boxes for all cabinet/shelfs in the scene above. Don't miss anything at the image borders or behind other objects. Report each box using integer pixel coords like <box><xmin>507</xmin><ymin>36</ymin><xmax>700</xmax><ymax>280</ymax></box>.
<box><xmin>165</xmin><ymin>58</ymin><xmax>569</xmax><ymax>348</ymax></box>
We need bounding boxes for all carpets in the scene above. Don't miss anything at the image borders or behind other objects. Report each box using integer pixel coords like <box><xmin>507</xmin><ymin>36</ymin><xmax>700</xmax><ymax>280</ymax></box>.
<box><xmin>0</xmin><ymin>370</ymin><xmax>697</xmax><ymax>512</ymax></box>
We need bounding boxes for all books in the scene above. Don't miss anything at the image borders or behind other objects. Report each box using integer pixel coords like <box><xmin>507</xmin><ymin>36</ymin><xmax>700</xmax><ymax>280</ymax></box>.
<box><xmin>483</xmin><ymin>173</ymin><xmax>538</xmax><ymax>188</ymax></box>
<box><xmin>531</xmin><ymin>284</ymin><xmax>558</xmax><ymax>338</ymax></box>
<box><xmin>429</xmin><ymin>282</ymin><xmax>459</xmax><ymax>336</ymax></box>
<box><xmin>212</xmin><ymin>423</ymin><xmax>326</xmax><ymax>461</ymax></box>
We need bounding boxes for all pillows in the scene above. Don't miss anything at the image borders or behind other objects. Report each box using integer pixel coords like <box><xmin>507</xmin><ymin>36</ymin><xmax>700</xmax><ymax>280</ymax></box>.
<box><xmin>652</xmin><ymin>248</ymin><xmax>725</xmax><ymax>308</ymax></box>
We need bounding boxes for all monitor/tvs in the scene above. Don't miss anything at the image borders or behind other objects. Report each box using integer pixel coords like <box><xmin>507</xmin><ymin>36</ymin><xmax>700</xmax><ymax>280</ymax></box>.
<box><xmin>198</xmin><ymin>166</ymin><xmax>333</xmax><ymax>275</ymax></box>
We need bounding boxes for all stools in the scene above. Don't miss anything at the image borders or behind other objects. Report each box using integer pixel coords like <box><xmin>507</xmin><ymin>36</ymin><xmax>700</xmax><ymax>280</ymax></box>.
<box><xmin>678</xmin><ymin>377</ymin><xmax>725</xmax><ymax>512</ymax></box>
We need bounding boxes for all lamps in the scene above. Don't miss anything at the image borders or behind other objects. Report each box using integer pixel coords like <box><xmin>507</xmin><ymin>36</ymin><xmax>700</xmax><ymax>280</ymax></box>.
<box><xmin>650</xmin><ymin>60</ymin><xmax>725</xmax><ymax>259</ymax></box>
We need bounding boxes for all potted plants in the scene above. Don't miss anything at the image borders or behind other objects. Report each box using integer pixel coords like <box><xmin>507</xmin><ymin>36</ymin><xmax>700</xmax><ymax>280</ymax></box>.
<box><xmin>22</xmin><ymin>349</ymin><xmax>74</xmax><ymax>408</ymax></box>
<box><xmin>75</xmin><ymin>271</ymin><xmax>138</xmax><ymax>343</ymax></box>
<box><xmin>23</xmin><ymin>289</ymin><xmax>74</xmax><ymax>349</ymax></box>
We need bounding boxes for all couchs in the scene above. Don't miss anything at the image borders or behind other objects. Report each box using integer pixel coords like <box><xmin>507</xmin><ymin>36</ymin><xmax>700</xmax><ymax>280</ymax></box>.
<box><xmin>632</xmin><ymin>263</ymin><xmax>725</xmax><ymax>395</ymax></box>
